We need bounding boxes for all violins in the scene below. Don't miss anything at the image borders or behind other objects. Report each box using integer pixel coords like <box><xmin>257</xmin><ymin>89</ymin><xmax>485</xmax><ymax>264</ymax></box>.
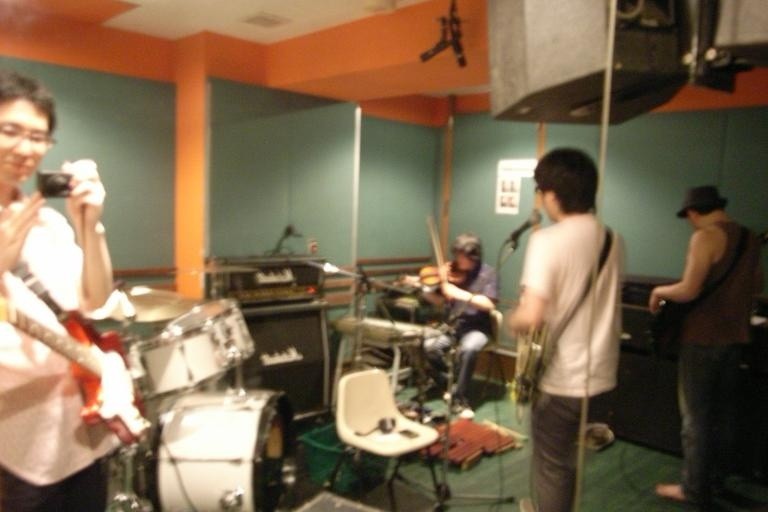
<box><xmin>392</xmin><ymin>259</ymin><xmax>469</xmax><ymax>290</ymax></box>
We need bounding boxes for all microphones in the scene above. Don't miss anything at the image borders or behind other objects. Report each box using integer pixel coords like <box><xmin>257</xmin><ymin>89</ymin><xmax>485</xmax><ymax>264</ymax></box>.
<box><xmin>507</xmin><ymin>212</ymin><xmax>541</xmax><ymax>241</ymax></box>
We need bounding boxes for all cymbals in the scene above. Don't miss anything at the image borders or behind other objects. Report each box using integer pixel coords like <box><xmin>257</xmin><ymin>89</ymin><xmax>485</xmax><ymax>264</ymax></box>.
<box><xmin>170</xmin><ymin>263</ymin><xmax>260</xmax><ymax>273</ymax></box>
<box><xmin>118</xmin><ymin>284</ymin><xmax>196</xmax><ymax>323</ymax></box>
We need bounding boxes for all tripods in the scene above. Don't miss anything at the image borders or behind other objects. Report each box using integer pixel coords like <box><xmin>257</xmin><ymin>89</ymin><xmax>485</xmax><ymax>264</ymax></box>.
<box><xmin>393</xmin><ymin>245</ymin><xmax>515</xmax><ymax>512</ymax></box>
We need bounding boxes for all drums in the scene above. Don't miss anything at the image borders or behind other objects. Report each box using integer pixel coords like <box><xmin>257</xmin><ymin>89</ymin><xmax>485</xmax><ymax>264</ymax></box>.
<box><xmin>122</xmin><ymin>296</ymin><xmax>258</xmax><ymax>402</ymax></box>
<box><xmin>149</xmin><ymin>387</ymin><xmax>296</xmax><ymax>512</ymax></box>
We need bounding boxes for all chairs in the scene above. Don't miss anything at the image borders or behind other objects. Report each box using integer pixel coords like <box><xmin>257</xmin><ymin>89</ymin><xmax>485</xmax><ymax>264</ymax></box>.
<box><xmin>329</xmin><ymin>369</ymin><xmax>441</xmax><ymax>512</ymax></box>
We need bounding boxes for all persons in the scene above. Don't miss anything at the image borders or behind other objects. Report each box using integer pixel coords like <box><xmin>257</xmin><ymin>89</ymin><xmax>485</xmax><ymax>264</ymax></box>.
<box><xmin>423</xmin><ymin>234</ymin><xmax>501</xmax><ymax>421</ymax></box>
<box><xmin>1</xmin><ymin>68</ymin><xmax>120</xmax><ymax>511</ymax></box>
<box><xmin>505</xmin><ymin>147</ymin><xmax>624</xmax><ymax>512</ymax></box>
<box><xmin>648</xmin><ymin>185</ymin><xmax>763</xmax><ymax>504</ymax></box>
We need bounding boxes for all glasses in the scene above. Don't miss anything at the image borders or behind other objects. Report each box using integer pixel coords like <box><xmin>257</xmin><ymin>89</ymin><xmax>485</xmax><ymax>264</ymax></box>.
<box><xmin>0</xmin><ymin>127</ymin><xmax>60</xmax><ymax>151</ymax></box>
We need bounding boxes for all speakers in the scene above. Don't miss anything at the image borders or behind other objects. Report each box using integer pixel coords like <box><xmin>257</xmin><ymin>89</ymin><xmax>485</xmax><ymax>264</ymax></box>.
<box><xmin>217</xmin><ymin>299</ymin><xmax>330</xmax><ymax>422</ymax></box>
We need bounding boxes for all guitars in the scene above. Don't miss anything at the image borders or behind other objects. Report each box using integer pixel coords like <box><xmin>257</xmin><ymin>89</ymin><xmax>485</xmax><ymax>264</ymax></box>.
<box><xmin>514</xmin><ymin>322</ymin><xmax>550</xmax><ymax>407</ymax></box>
<box><xmin>0</xmin><ymin>299</ymin><xmax>152</xmax><ymax>448</ymax></box>
<box><xmin>647</xmin><ymin>230</ymin><xmax>767</xmax><ymax>361</ymax></box>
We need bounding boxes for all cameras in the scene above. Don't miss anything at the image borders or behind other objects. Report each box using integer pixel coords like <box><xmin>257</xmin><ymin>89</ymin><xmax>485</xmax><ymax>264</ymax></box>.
<box><xmin>38</xmin><ymin>171</ymin><xmax>74</xmax><ymax>197</ymax></box>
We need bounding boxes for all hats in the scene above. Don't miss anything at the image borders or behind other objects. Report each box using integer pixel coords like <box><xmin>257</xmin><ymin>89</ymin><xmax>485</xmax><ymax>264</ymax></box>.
<box><xmin>674</xmin><ymin>183</ymin><xmax>728</xmax><ymax>218</ymax></box>
